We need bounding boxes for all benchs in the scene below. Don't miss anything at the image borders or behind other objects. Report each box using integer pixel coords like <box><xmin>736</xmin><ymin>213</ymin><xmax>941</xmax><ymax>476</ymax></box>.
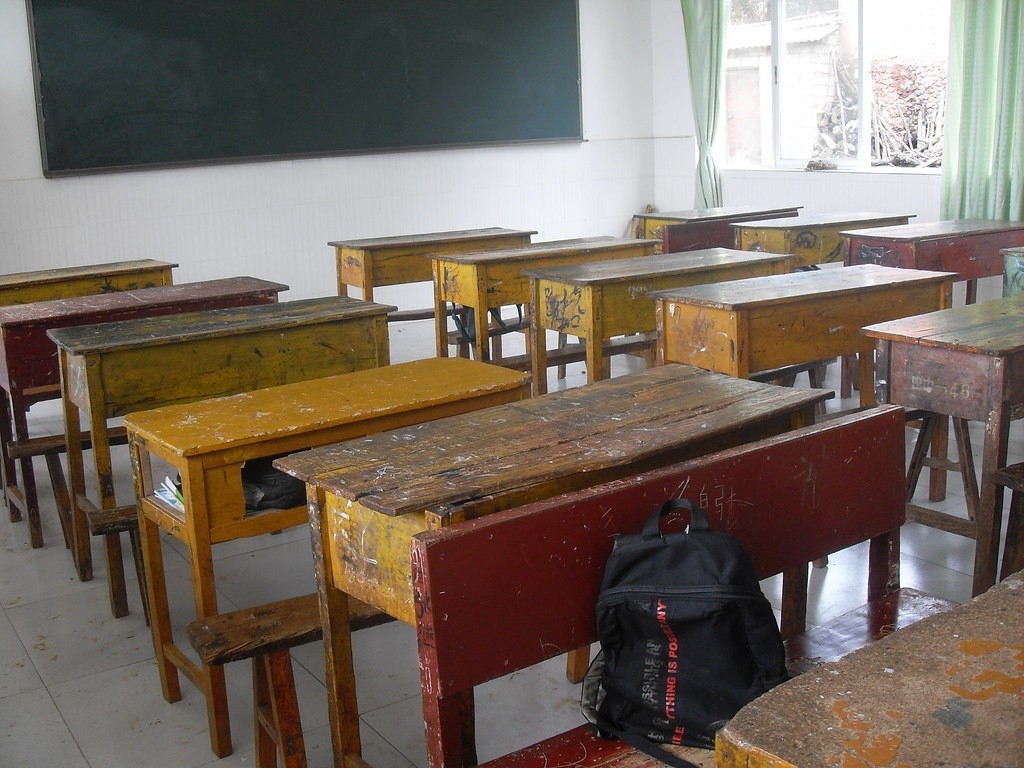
<box><xmin>85</xmin><ymin>498</ymin><xmax>152</xmax><ymax>629</ymax></box>
<box><xmin>494</xmin><ymin>330</ymin><xmax>657</xmax><ymax>374</ymax></box>
<box><xmin>385</xmin><ymin>305</ymin><xmax>502</xmax><ymax>361</ymax></box>
<box><xmin>7</xmin><ymin>423</ymin><xmax>128</xmax><ymax>569</ymax></box>
<box><xmin>750</xmin><ymin>356</ymin><xmax>837</xmax><ymax>415</ymax></box>
<box><xmin>446</xmin><ymin>313</ymin><xmax>569</xmax><ymax>380</ymax></box>
<box><xmin>185</xmin><ymin>590</ymin><xmax>396</xmax><ymax>766</ymax></box>
<box><xmin>813</xmin><ymin>401</ymin><xmax>979</xmax><ymax>523</ymax></box>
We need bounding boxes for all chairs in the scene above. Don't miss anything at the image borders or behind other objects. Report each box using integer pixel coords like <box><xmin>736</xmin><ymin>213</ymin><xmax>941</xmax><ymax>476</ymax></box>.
<box><xmin>409</xmin><ymin>402</ymin><xmax>956</xmax><ymax>768</ymax></box>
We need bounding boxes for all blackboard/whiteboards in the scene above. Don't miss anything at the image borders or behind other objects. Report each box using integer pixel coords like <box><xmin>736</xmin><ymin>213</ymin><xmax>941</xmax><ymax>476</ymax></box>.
<box><xmin>25</xmin><ymin>1</ymin><xmax>589</xmax><ymax>181</ymax></box>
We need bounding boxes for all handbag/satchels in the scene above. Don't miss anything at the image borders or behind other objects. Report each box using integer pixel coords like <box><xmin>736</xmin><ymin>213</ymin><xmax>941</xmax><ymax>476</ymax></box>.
<box><xmin>241</xmin><ymin>451</ymin><xmax>305</xmax><ymax>509</ymax></box>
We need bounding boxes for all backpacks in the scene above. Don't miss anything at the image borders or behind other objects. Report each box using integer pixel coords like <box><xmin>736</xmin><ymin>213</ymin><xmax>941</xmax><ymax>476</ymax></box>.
<box><xmin>580</xmin><ymin>499</ymin><xmax>787</xmax><ymax>749</ymax></box>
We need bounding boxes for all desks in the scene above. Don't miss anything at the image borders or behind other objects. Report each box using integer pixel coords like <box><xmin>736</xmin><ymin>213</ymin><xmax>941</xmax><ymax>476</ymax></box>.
<box><xmin>860</xmin><ymin>291</ymin><xmax>1024</xmax><ymax>604</ymax></box>
<box><xmin>271</xmin><ymin>361</ymin><xmax>836</xmax><ymax>767</ymax></box>
<box><xmin>729</xmin><ymin>212</ymin><xmax>917</xmax><ymax>273</ymax></box>
<box><xmin>519</xmin><ymin>245</ymin><xmax>794</xmax><ymax>399</ymax></box>
<box><xmin>634</xmin><ymin>202</ymin><xmax>805</xmax><ymax>257</ymax></box>
<box><xmin>326</xmin><ymin>227</ymin><xmax>538</xmax><ymax>300</ymax></box>
<box><xmin>716</xmin><ymin>570</ymin><xmax>1023</xmax><ymax>767</ymax></box>
<box><xmin>837</xmin><ymin>217</ymin><xmax>1024</xmax><ymax>305</ymax></box>
<box><xmin>1</xmin><ymin>275</ymin><xmax>292</xmax><ymax>548</ymax></box>
<box><xmin>430</xmin><ymin>235</ymin><xmax>663</xmax><ymax>363</ymax></box>
<box><xmin>0</xmin><ymin>256</ymin><xmax>181</xmax><ymax>308</ymax></box>
<box><xmin>649</xmin><ymin>262</ymin><xmax>959</xmax><ymax>433</ymax></box>
<box><xmin>47</xmin><ymin>293</ymin><xmax>397</xmax><ymax>617</ymax></box>
<box><xmin>123</xmin><ymin>353</ymin><xmax>532</xmax><ymax>759</ymax></box>
